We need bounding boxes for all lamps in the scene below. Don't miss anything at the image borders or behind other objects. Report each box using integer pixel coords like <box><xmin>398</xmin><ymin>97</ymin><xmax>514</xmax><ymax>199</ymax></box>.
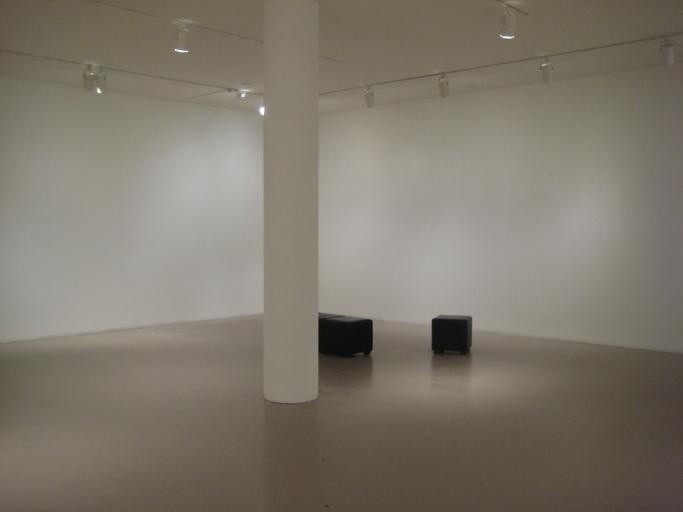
<box><xmin>498</xmin><ymin>6</ymin><xmax>517</xmax><ymax>40</ymax></box>
<box><xmin>659</xmin><ymin>36</ymin><xmax>678</xmax><ymax>65</ymax></box>
<box><xmin>173</xmin><ymin>24</ymin><xmax>192</xmax><ymax>53</ymax></box>
<box><xmin>438</xmin><ymin>76</ymin><xmax>449</xmax><ymax>98</ymax></box>
<box><xmin>363</xmin><ymin>88</ymin><xmax>375</xmax><ymax>108</ymax></box>
<box><xmin>539</xmin><ymin>62</ymin><xmax>553</xmax><ymax>82</ymax></box>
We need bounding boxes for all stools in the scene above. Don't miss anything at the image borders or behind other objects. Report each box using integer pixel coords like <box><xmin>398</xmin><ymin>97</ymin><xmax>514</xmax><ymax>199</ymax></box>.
<box><xmin>431</xmin><ymin>315</ymin><xmax>472</xmax><ymax>353</ymax></box>
<box><xmin>319</xmin><ymin>312</ymin><xmax>344</xmax><ymax>330</ymax></box>
<box><xmin>319</xmin><ymin>316</ymin><xmax>372</xmax><ymax>357</ymax></box>
